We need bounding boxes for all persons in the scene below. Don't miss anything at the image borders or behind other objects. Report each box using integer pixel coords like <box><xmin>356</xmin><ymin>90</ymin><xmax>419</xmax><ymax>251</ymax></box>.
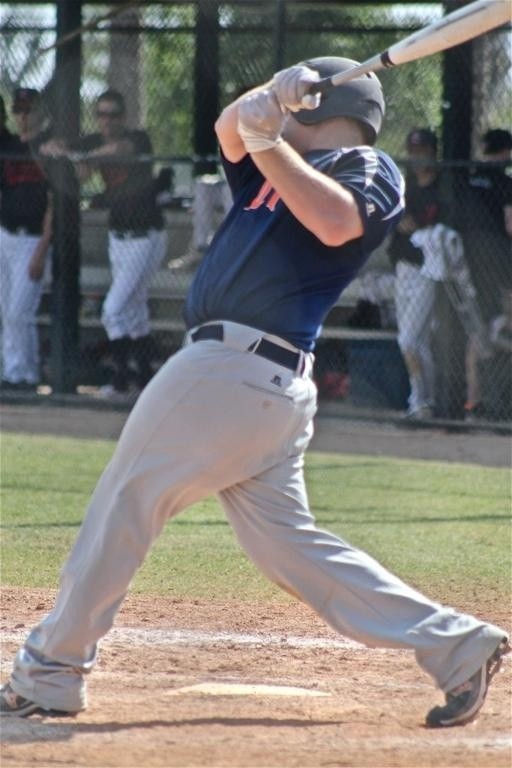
<box><xmin>1</xmin><ymin>86</ymin><xmax>57</xmax><ymax>403</ymax></box>
<box><xmin>376</xmin><ymin>128</ymin><xmax>454</xmax><ymax>424</ymax></box>
<box><xmin>425</xmin><ymin>127</ymin><xmax>511</xmax><ymax>424</ymax></box>
<box><xmin>1</xmin><ymin>53</ymin><xmax>511</xmax><ymax>731</ymax></box>
<box><xmin>153</xmin><ymin>158</ymin><xmax>235</xmax><ymax>270</ymax></box>
<box><xmin>39</xmin><ymin>87</ymin><xmax>171</xmax><ymax>405</ymax></box>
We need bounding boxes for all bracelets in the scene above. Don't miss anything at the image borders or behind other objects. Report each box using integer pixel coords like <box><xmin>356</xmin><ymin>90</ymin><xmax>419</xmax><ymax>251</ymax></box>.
<box><xmin>76</xmin><ymin>150</ymin><xmax>89</xmax><ymax>163</ymax></box>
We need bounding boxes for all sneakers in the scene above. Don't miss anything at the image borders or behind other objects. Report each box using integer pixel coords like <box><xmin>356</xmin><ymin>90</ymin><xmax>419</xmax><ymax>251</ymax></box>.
<box><xmin>0</xmin><ymin>680</ymin><xmax>88</xmax><ymax>718</ymax></box>
<box><xmin>423</xmin><ymin>631</ymin><xmax>512</xmax><ymax>727</ymax></box>
<box><xmin>88</xmin><ymin>383</ymin><xmax>130</xmax><ymax>403</ymax></box>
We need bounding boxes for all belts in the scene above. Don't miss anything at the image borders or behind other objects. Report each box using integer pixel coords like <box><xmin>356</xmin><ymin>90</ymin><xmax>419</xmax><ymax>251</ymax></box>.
<box><xmin>2</xmin><ymin>224</ymin><xmax>43</xmax><ymax>238</ymax></box>
<box><xmin>111</xmin><ymin>228</ymin><xmax>147</xmax><ymax>240</ymax></box>
<box><xmin>184</xmin><ymin>321</ymin><xmax>309</xmax><ymax>379</ymax></box>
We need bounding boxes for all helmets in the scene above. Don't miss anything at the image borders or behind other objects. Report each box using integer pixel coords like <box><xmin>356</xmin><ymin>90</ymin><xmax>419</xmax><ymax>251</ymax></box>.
<box><xmin>287</xmin><ymin>54</ymin><xmax>390</xmax><ymax>147</ymax></box>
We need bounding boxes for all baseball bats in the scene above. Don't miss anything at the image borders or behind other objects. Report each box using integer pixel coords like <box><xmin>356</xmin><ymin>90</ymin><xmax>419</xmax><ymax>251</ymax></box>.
<box><xmin>310</xmin><ymin>0</ymin><xmax>512</xmax><ymax>94</ymax></box>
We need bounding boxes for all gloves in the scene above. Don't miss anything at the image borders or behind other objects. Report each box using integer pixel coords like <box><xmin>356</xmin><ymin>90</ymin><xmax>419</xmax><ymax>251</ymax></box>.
<box><xmin>236</xmin><ymin>64</ymin><xmax>324</xmax><ymax>154</ymax></box>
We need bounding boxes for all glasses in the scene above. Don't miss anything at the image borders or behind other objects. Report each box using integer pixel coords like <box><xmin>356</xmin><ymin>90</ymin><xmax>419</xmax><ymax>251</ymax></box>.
<box><xmin>11</xmin><ymin>106</ymin><xmax>32</xmax><ymax>116</ymax></box>
<box><xmin>96</xmin><ymin>109</ymin><xmax>124</xmax><ymax>119</ymax></box>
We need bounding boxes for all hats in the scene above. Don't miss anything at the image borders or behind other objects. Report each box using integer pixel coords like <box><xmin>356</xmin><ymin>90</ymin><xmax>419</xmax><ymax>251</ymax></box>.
<box><xmin>403</xmin><ymin>126</ymin><xmax>441</xmax><ymax>150</ymax></box>
<box><xmin>12</xmin><ymin>85</ymin><xmax>42</xmax><ymax>110</ymax></box>
